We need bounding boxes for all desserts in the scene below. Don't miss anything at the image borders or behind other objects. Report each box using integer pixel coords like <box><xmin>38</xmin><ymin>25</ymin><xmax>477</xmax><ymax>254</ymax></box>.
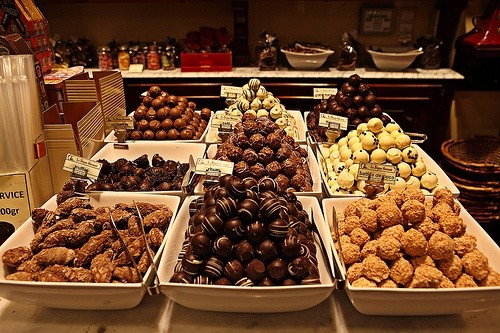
<box><xmin>2</xmin><ymin>74</ymin><xmax>500</xmax><ymax>288</ymax></box>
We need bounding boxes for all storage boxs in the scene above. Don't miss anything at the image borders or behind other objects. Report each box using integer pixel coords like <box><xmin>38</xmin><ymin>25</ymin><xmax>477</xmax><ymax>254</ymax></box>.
<box><xmin>64</xmin><ymin>71</ymin><xmax>127</xmax><ymax>137</ymax></box>
<box><xmin>41</xmin><ymin>101</ymin><xmax>106</xmax><ymax>195</ymax></box>
<box><xmin>0</xmin><ymin>155</ymin><xmax>53</xmax><ymax>246</ymax></box>
<box><xmin>180</xmin><ymin>49</ymin><xmax>233</xmax><ymax>72</ymax></box>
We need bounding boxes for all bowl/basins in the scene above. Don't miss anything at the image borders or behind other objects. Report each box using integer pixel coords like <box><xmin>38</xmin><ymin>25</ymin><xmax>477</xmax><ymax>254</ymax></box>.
<box><xmin>0</xmin><ymin>109</ymin><xmax>500</xmax><ymax>317</ymax></box>
<box><xmin>279</xmin><ymin>45</ymin><xmax>334</xmax><ymax>69</ymax></box>
<box><xmin>365</xmin><ymin>45</ymin><xmax>424</xmax><ymax>72</ymax></box>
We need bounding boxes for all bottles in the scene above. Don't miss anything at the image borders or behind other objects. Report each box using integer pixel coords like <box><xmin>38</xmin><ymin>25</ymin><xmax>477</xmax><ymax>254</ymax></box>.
<box><xmin>97</xmin><ymin>40</ymin><xmax>180</xmax><ymax>70</ymax></box>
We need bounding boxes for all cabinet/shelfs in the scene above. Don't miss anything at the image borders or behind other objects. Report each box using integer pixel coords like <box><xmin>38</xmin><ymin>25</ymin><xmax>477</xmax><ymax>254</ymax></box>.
<box><xmin>123</xmin><ymin>79</ymin><xmax>460</xmax><ymax>157</ymax></box>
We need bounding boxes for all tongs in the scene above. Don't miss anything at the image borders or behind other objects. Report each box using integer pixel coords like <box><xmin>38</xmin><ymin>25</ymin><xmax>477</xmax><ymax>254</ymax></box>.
<box><xmin>404</xmin><ymin>131</ymin><xmax>428</xmax><ymax>144</ymax></box>
<box><xmin>295</xmin><ymin>43</ymin><xmax>330</xmax><ymax>52</ymax></box>
<box><xmin>181</xmin><ymin>151</ymin><xmax>209</xmax><ymax>195</ymax></box>
<box><xmin>310</xmin><ymin>206</ymin><xmax>348</xmax><ymax>292</ymax></box>
<box><xmin>107</xmin><ymin>199</ymin><xmax>161</xmax><ymax>297</ymax></box>
